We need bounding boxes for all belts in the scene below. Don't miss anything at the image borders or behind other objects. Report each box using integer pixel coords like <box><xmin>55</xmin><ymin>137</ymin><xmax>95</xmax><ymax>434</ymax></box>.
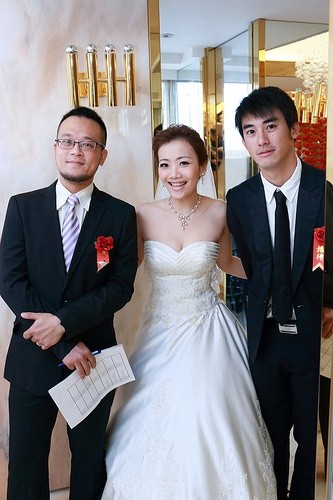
<box><xmin>263</xmin><ymin>322</ymin><xmax>299</xmax><ymax>335</ymax></box>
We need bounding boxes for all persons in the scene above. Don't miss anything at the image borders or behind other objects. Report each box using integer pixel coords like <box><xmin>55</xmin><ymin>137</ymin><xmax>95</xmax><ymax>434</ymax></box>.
<box><xmin>154</xmin><ymin>123</ymin><xmax>223</xmax><ymax>171</ymax></box>
<box><xmin>99</xmin><ymin>123</ymin><xmax>279</xmax><ymax>500</ymax></box>
<box><xmin>318</xmin><ymin>180</ymin><xmax>333</xmax><ymax>479</ymax></box>
<box><xmin>226</xmin><ymin>87</ymin><xmax>325</xmax><ymax>500</ymax></box>
<box><xmin>0</xmin><ymin>107</ymin><xmax>138</xmax><ymax>500</ymax></box>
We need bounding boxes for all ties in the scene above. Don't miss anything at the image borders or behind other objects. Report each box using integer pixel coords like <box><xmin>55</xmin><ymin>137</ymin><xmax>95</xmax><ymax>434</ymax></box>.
<box><xmin>61</xmin><ymin>194</ymin><xmax>80</xmax><ymax>273</ymax></box>
<box><xmin>271</xmin><ymin>190</ymin><xmax>292</xmax><ymax>323</ymax></box>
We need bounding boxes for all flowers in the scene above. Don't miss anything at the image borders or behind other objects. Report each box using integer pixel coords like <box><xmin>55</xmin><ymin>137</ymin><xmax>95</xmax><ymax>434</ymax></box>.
<box><xmin>313</xmin><ymin>226</ymin><xmax>325</xmax><ymax>271</ymax></box>
<box><xmin>94</xmin><ymin>236</ymin><xmax>113</xmax><ymax>272</ymax></box>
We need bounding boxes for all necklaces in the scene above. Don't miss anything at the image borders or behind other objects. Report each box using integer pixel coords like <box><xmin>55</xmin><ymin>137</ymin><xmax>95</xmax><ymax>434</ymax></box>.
<box><xmin>168</xmin><ymin>193</ymin><xmax>201</xmax><ymax>230</ymax></box>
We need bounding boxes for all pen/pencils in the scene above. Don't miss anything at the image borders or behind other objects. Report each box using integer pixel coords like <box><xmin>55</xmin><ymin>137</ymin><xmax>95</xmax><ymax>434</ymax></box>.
<box><xmin>58</xmin><ymin>350</ymin><xmax>101</xmax><ymax>367</ymax></box>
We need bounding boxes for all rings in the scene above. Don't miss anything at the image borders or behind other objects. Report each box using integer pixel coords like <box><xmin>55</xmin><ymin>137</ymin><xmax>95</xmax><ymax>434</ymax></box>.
<box><xmin>36</xmin><ymin>341</ymin><xmax>41</xmax><ymax>346</ymax></box>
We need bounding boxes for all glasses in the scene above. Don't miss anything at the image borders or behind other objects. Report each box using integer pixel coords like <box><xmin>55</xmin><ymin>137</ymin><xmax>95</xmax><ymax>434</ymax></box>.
<box><xmin>56</xmin><ymin>139</ymin><xmax>102</xmax><ymax>151</ymax></box>
